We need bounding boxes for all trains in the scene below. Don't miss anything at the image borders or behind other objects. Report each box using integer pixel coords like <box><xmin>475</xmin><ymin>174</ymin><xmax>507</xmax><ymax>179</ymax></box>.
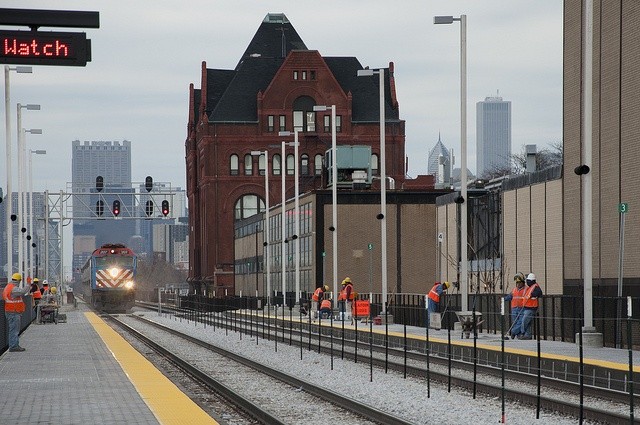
<box><xmin>80</xmin><ymin>243</ymin><xmax>138</xmax><ymax>313</ymax></box>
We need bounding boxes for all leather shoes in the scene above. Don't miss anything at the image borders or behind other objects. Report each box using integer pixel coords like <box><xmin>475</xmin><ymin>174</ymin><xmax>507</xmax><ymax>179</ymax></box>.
<box><xmin>10</xmin><ymin>347</ymin><xmax>25</xmax><ymax>351</ymax></box>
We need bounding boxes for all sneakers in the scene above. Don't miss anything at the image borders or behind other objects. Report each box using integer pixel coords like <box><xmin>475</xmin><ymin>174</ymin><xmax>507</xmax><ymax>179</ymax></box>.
<box><xmin>511</xmin><ymin>332</ymin><xmax>515</xmax><ymax>339</ymax></box>
<box><xmin>520</xmin><ymin>335</ymin><xmax>532</xmax><ymax>340</ymax></box>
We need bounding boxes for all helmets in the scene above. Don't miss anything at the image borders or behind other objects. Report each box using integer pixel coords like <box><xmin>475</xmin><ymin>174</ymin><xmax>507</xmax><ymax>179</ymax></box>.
<box><xmin>324</xmin><ymin>285</ymin><xmax>330</xmax><ymax>291</ymax></box>
<box><xmin>33</xmin><ymin>278</ymin><xmax>39</xmax><ymax>281</ymax></box>
<box><xmin>516</xmin><ymin>278</ymin><xmax>522</xmax><ymax>282</ymax></box>
<box><xmin>42</xmin><ymin>280</ymin><xmax>48</xmax><ymax>284</ymax></box>
<box><xmin>12</xmin><ymin>273</ymin><xmax>21</xmax><ymax>279</ymax></box>
<box><xmin>341</xmin><ymin>280</ymin><xmax>347</xmax><ymax>284</ymax></box>
<box><xmin>445</xmin><ymin>282</ymin><xmax>450</xmax><ymax>289</ymax></box>
<box><xmin>51</xmin><ymin>286</ymin><xmax>57</xmax><ymax>293</ymax></box>
<box><xmin>345</xmin><ymin>277</ymin><xmax>350</xmax><ymax>282</ymax></box>
<box><xmin>526</xmin><ymin>272</ymin><xmax>536</xmax><ymax>280</ymax></box>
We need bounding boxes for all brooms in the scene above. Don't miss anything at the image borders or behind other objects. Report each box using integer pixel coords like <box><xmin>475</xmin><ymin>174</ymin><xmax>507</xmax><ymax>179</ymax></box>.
<box><xmin>505</xmin><ymin>298</ymin><xmax>530</xmax><ymax>340</ymax></box>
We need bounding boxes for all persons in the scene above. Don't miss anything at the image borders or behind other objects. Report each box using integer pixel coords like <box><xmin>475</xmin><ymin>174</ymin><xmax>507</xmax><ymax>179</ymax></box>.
<box><xmin>338</xmin><ymin>280</ymin><xmax>346</xmax><ymax>313</ymax></box>
<box><xmin>428</xmin><ymin>281</ymin><xmax>451</xmax><ymax>330</ymax></box>
<box><xmin>31</xmin><ymin>277</ymin><xmax>57</xmax><ymax>321</ymax></box>
<box><xmin>311</xmin><ymin>284</ymin><xmax>329</xmax><ymax>318</ymax></box>
<box><xmin>3</xmin><ymin>272</ymin><xmax>31</xmax><ymax>353</ymax></box>
<box><xmin>504</xmin><ymin>272</ymin><xmax>527</xmax><ymax>340</ymax></box>
<box><xmin>343</xmin><ymin>277</ymin><xmax>354</xmax><ymax>319</ymax></box>
<box><xmin>519</xmin><ymin>272</ymin><xmax>543</xmax><ymax>339</ymax></box>
<box><xmin>320</xmin><ymin>299</ymin><xmax>331</xmax><ymax>319</ymax></box>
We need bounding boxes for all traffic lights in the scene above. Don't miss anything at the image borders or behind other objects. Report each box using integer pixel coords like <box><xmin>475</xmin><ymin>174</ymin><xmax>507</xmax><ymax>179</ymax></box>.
<box><xmin>162</xmin><ymin>200</ymin><xmax>170</xmax><ymax>217</ymax></box>
<box><xmin>113</xmin><ymin>200</ymin><xmax>120</xmax><ymax>216</ymax></box>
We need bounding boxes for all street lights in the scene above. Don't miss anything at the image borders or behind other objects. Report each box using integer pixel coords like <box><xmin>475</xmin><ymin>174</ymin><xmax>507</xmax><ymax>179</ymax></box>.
<box><xmin>17</xmin><ymin>103</ymin><xmax>40</xmax><ymax>289</ymax></box>
<box><xmin>433</xmin><ymin>14</ymin><xmax>482</xmax><ymax>333</ymax></box>
<box><xmin>357</xmin><ymin>68</ymin><xmax>394</xmax><ymax>325</ymax></box>
<box><xmin>313</xmin><ymin>104</ymin><xmax>339</xmax><ymax>317</ymax></box>
<box><xmin>4</xmin><ymin>65</ymin><xmax>32</xmax><ymax>284</ymax></box>
<box><xmin>251</xmin><ymin>149</ymin><xmax>274</xmax><ymax>315</ymax></box>
<box><xmin>279</xmin><ymin>131</ymin><xmax>301</xmax><ymax>317</ymax></box>
<box><xmin>23</xmin><ymin>128</ymin><xmax>43</xmax><ymax>288</ymax></box>
<box><xmin>28</xmin><ymin>149</ymin><xmax>46</xmax><ymax>286</ymax></box>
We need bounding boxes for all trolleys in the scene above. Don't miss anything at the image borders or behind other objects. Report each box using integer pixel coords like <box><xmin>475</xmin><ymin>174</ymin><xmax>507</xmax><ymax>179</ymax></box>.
<box><xmin>455</xmin><ymin>311</ymin><xmax>486</xmax><ymax>340</ymax></box>
